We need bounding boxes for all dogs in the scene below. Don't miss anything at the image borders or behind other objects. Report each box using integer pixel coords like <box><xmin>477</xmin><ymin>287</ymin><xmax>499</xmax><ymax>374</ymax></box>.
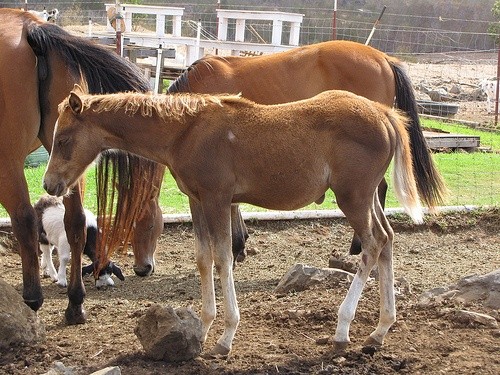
<box><xmin>33</xmin><ymin>193</ymin><xmax>125</xmax><ymax>291</ymax></box>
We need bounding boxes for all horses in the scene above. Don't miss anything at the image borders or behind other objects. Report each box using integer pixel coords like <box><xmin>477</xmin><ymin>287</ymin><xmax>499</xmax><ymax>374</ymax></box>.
<box><xmin>42</xmin><ymin>82</ymin><xmax>426</xmax><ymax>360</ymax></box>
<box><xmin>0</xmin><ymin>6</ymin><xmax>161</xmax><ymax>326</ymax></box>
<box><xmin>110</xmin><ymin>40</ymin><xmax>450</xmax><ymax>278</ymax></box>
